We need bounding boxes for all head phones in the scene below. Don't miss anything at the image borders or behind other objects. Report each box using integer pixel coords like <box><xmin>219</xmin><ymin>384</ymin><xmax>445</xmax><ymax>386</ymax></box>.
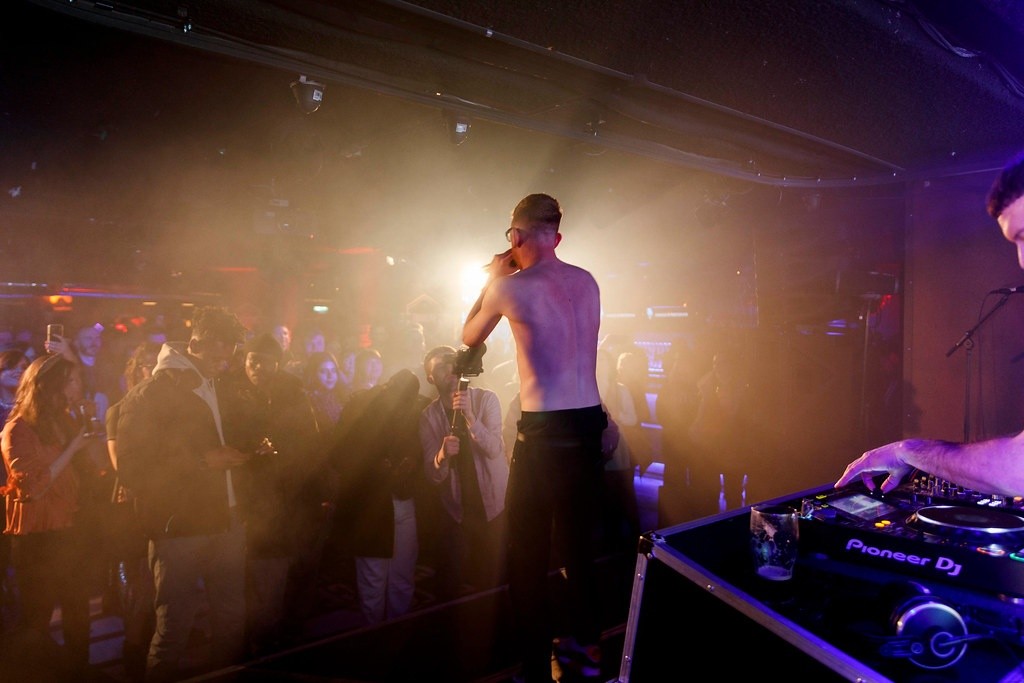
<box><xmin>844</xmin><ymin>579</ymin><xmax>968</xmax><ymax>670</ymax></box>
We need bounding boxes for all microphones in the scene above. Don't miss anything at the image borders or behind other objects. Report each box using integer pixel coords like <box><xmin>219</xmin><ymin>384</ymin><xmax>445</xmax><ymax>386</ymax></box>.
<box><xmin>991</xmin><ymin>285</ymin><xmax>1024</xmax><ymax>294</ymax></box>
<box><xmin>482</xmin><ymin>260</ymin><xmax>516</xmax><ymax>273</ymax></box>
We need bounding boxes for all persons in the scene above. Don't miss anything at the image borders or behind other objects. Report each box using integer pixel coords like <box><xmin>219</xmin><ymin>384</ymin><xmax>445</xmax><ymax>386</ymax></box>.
<box><xmin>833</xmin><ymin>161</ymin><xmax>1024</xmax><ymax>498</ymax></box>
<box><xmin>461</xmin><ymin>193</ymin><xmax>633</xmax><ymax>683</ymax></box>
<box><xmin>0</xmin><ymin>302</ymin><xmax>765</xmax><ymax>683</ymax></box>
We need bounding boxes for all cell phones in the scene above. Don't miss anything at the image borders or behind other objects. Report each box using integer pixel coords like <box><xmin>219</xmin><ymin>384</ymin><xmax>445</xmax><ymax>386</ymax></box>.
<box><xmin>47</xmin><ymin>324</ymin><xmax>63</xmax><ymax>353</ymax></box>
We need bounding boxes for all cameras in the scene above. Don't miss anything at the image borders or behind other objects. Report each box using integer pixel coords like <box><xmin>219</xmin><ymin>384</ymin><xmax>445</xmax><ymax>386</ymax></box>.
<box><xmin>451</xmin><ymin>343</ymin><xmax>486</xmax><ymax>374</ymax></box>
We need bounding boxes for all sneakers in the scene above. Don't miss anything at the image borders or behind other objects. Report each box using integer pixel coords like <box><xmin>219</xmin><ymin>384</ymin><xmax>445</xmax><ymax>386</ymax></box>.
<box><xmin>553</xmin><ymin>636</ymin><xmax>601</xmax><ymax>678</ymax></box>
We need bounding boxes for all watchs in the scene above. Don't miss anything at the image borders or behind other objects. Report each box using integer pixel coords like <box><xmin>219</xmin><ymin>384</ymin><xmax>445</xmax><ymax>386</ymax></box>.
<box><xmin>197</xmin><ymin>452</ymin><xmax>210</xmax><ymax>474</ymax></box>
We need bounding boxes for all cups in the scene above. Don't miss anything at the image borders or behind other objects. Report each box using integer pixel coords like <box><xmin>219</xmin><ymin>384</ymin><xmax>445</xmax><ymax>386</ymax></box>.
<box><xmin>76</xmin><ymin>400</ymin><xmax>98</xmax><ymax>438</ymax></box>
<box><xmin>750</xmin><ymin>505</ymin><xmax>799</xmax><ymax>580</ymax></box>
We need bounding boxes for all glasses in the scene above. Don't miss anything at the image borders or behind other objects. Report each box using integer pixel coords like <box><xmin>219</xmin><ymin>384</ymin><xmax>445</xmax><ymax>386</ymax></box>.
<box><xmin>505</xmin><ymin>228</ymin><xmax>513</xmax><ymax>242</ymax></box>
